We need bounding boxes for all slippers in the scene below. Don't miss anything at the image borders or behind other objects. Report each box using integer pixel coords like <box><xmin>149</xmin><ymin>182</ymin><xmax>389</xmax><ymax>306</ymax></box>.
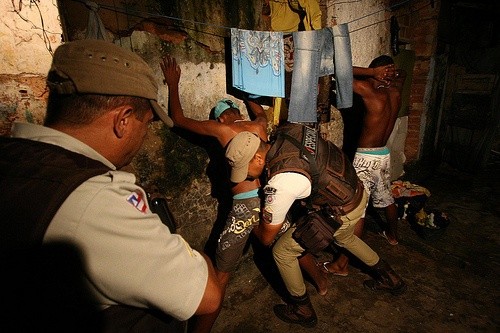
<box><xmin>321</xmin><ymin>261</ymin><xmax>348</xmax><ymax>276</ymax></box>
<box><xmin>378</xmin><ymin>230</ymin><xmax>399</xmax><ymax>246</ymax></box>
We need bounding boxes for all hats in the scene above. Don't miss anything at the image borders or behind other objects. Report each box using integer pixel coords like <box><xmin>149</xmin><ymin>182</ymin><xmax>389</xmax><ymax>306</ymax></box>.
<box><xmin>368</xmin><ymin>56</ymin><xmax>394</xmax><ymax>68</ymax></box>
<box><xmin>215</xmin><ymin>99</ymin><xmax>240</xmax><ymax>120</ymax></box>
<box><xmin>45</xmin><ymin>38</ymin><xmax>174</xmax><ymax>127</ymax></box>
<box><xmin>225</xmin><ymin>131</ymin><xmax>261</xmax><ymax>183</ymax></box>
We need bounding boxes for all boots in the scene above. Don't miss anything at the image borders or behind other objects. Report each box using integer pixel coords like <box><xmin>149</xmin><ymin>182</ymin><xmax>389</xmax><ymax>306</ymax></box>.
<box><xmin>273</xmin><ymin>291</ymin><xmax>317</xmax><ymax>327</ymax></box>
<box><xmin>364</xmin><ymin>259</ymin><xmax>407</xmax><ymax>294</ymax></box>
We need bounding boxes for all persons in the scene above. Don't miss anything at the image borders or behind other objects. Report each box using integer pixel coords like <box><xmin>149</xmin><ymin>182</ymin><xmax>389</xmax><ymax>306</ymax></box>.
<box><xmin>226</xmin><ymin>123</ymin><xmax>406</xmax><ymax>327</ymax></box>
<box><xmin>316</xmin><ymin>56</ymin><xmax>407</xmax><ymax>276</ymax></box>
<box><xmin>0</xmin><ymin>38</ymin><xmax>221</xmax><ymax>333</ymax></box>
<box><xmin>159</xmin><ymin>56</ymin><xmax>328</xmax><ymax>333</ymax></box>
